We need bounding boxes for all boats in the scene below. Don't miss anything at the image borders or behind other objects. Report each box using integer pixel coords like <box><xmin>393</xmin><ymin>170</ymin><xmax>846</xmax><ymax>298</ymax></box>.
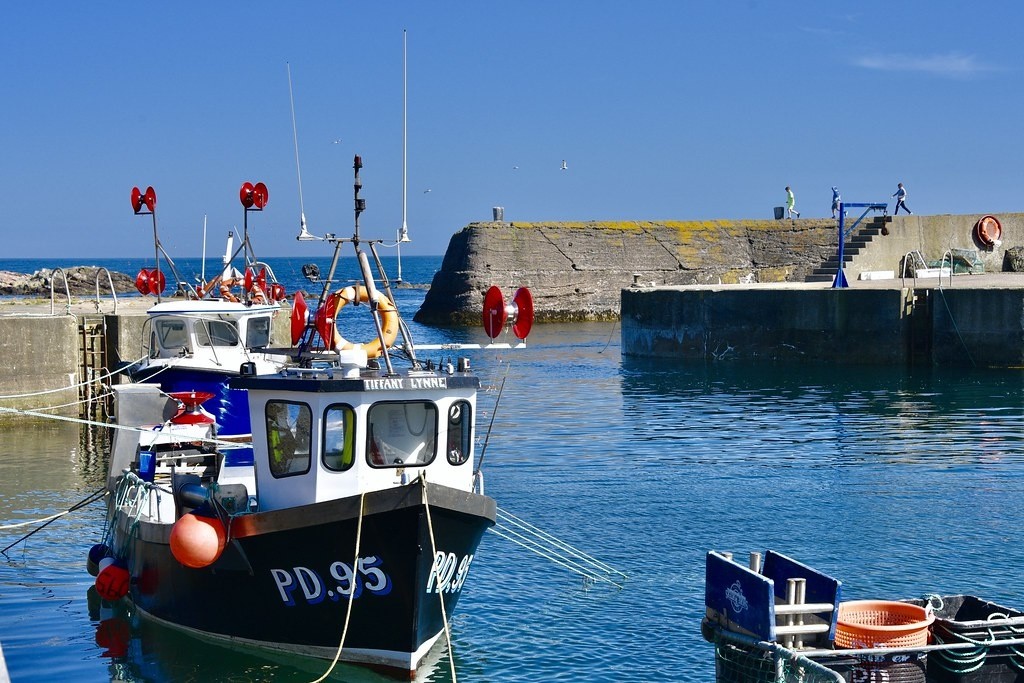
<box><xmin>83</xmin><ymin>26</ymin><xmax>501</xmax><ymax>683</ymax></box>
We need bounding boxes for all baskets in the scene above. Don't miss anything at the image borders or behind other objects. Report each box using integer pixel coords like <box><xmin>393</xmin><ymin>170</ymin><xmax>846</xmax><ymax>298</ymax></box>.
<box><xmin>835</xmin><ymin>599</ymin><xmax>930</xmax><ymax>648</ymax></box>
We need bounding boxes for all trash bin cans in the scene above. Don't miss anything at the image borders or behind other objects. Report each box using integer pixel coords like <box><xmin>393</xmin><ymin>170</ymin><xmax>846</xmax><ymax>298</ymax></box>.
<box><xmin>774</xmin><ymin>207</ymin><xmax>784</xmax><ymax>220</ymax></box>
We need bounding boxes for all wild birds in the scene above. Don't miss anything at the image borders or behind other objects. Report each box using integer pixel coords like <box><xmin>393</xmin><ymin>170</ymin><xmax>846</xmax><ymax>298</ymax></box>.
<box><xmin>332</xmin><ymin>140</ymin><xmax>342</xmax><ymax>144</ymax></box>
<box><xmin>423</xmin><ymin>189</ymin><xmax>432</xmax><ymax>193</ymax></box>
<box><xmin>513</xmin><ymin>166</ymin><xmax>518</xmax><ymax>169</ymax></box>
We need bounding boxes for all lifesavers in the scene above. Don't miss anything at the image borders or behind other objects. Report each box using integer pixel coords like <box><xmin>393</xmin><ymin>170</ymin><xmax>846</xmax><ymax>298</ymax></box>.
<box><xmin>319</xmin><ymin>285</ymin><xmax>401</xmax><ymax>359</ymax></box>
<box><xmin>981</xmin><ymin>217</ymin><xmax>999</xmax><ymax>243</ymax></box>
<box><xmin>193</xmin><ymin>274</ymin><xmax>221</xmax><ymax>300</ymax></box>
<box><xmin>220</xmin><ymin>278</ymin><xmax>264</xmax><ymax>304</ymax></box>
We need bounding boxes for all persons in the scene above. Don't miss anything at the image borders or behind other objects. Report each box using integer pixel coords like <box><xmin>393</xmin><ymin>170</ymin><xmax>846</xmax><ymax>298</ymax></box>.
<box><xmin>784</xmin><ymin>186</ymin><xmax>800</xmax><ymax>219</ymax></box>
<box><xmin>830</xmin><ymin>185</ymin><xmax>848</xmax><ymax>218</ymax></box>
<box><xmin>891</xmin><ymin>182</ymin><xmax>913</xmax><ymax>215</ymax></box>
